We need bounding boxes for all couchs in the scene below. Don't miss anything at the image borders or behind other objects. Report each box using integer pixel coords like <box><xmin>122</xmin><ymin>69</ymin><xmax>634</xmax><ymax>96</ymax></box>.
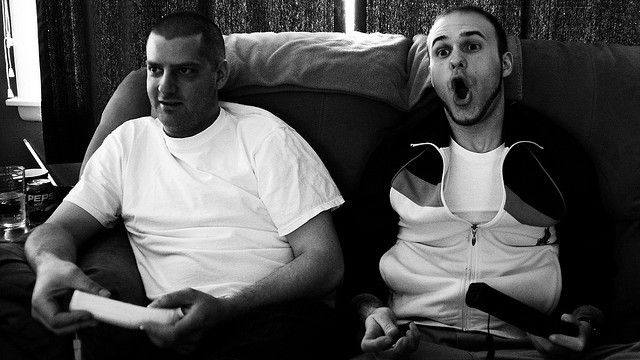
<box><xmin>78</xmin><ymin>31</ymin><xmax>640</xmax><ymax>360</ymax></box>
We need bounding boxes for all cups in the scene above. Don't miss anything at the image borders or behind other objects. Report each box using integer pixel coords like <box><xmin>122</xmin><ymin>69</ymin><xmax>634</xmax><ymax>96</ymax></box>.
<box><xmin>0</xmin><ymin>167</ymin><xmax>27</xmax><ymax>234</ymax></box>
<box><xmin>26</xmin><ymin>168</ymin><xmax>48</xmax><ymax>179</ymax></box>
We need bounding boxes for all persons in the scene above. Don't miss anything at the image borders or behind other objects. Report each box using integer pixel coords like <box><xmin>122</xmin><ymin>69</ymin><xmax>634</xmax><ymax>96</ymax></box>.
<box><xmin>345</xmin><ymin>7</ymin><xmax>620</xmax><ymax>360</ymax></box>
<box><xmin>23</xmin><ymin>13</ymin><xmax>345</xmax><ymax>359</ymax></box>
